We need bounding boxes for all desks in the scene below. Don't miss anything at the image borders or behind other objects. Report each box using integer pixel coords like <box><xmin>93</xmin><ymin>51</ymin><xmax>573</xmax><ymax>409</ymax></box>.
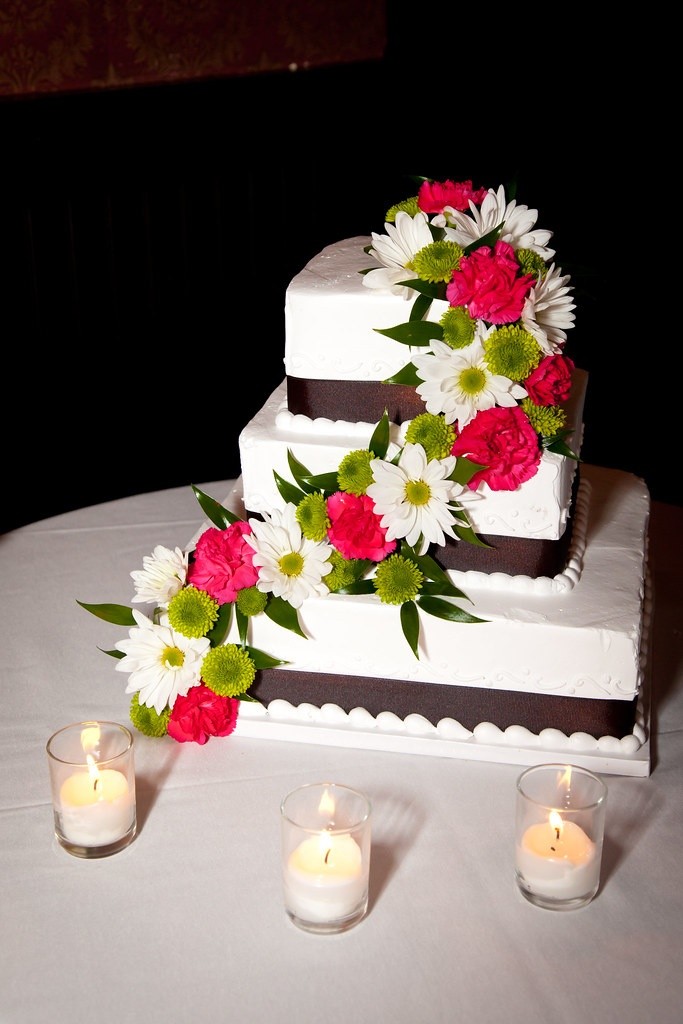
<box><xmin>1</xmin><ymin>480</ymin><xmax>683</xmax><ymax>1024</ymax></box>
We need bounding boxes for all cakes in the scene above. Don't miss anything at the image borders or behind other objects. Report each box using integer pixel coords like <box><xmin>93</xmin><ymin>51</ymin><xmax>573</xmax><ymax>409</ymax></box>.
<box><xmin>76</xmin><ymin>183</ymin><xmax>651</xmax><ymax>754</ymax></box>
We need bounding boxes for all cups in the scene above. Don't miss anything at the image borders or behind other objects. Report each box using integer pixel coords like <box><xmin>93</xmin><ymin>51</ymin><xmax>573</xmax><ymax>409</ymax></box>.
<box><xmin>280</xmin><ymin>782</ymin><xmax>373</xmax><ymax>937</ymax></box>
<box><xmin>512</xmin><ymin>762</ymin><xmax>609</xmax><ymax>911</ymax></box>
<box><xmin>46</xmin><ymin>720</ymin><xmax>138</xmax><ymax>860</ymax></box>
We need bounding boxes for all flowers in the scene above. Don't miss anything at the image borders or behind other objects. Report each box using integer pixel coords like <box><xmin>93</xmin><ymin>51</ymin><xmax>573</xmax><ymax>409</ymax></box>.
<box><xmin>76</xmin><ymin>180</ymin><xmax>577</xmax><ymax>744</ymax></box>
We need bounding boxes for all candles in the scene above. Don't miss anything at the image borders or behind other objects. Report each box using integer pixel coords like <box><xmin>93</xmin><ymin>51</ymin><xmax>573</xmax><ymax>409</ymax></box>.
<box><xmin>58</xmin><ymin>756</ymin><xmax>135</xmax><ymax>847</ymax></box>
<box><xmin>286</xmin><ymin>833</ymin><xmax>366</xmax><ymax>925</ymax></box>
<box><xmin>517</xmin><ymin>810</ymin><xmax>599</xmax><ymax>900</ymax></box>
